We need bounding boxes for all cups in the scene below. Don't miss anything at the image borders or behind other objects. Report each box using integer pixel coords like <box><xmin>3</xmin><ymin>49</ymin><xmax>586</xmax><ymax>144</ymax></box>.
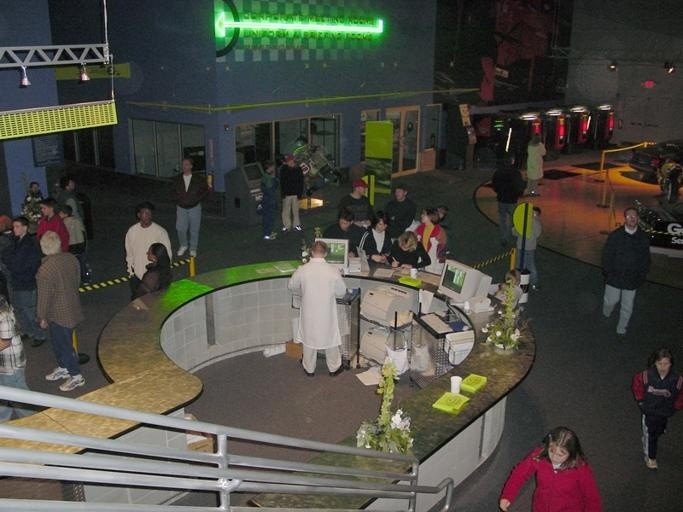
<box><xmin>451</xmin><ymin>376</ymin><xmax>463</xmax><ymax>394</ymax></box>
<box><xmin>410</xmin><ymin>268</ymin><xmax>418</xmax><ymax>278</ymax></box>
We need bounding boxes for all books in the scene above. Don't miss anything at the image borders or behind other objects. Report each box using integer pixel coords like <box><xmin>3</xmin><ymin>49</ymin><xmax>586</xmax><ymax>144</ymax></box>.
<box><xmin>446</xmin><ymin>329</ymin><xmax>475</xmax><ymax>365</ymax></box>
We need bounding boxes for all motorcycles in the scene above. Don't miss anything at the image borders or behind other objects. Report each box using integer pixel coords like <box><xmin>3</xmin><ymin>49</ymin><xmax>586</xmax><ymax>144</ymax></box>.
<box><xmin>652</xmin><ymin>159</ymin><xmax>681</xmax><ymax>206</ymax></box>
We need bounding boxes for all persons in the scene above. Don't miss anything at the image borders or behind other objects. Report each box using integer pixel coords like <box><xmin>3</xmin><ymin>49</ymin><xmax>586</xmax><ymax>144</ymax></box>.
<box><xmin>600</xmin><ymin>208</ymin><xmax>652</xmax><ymax>342</ymax></box>
<box><xmin>260</xmin><ymin>160</ymin><xmax>278</xmax><ymax>240</ymax></box>
<box><xmin>288</xmin><ymin>241</ymin><xmax>346</xmax><ymax>377</ymax></box>
<box><xmin>126</xmin><ymin>201</ymin><xmax>173</xmax><ymax>301</ymax></box>
<box><xmin>499</xmin><ymin>427</ymin><xmax>602</xmax><ymax>512</ymax></box>
<box><xmin>279</xmin><ymin>154</ymin><xmax>303</xmax><ymax>232</ymax></box>
<box><xmin>0</xmin><ymin>176</ymin><xmax>86</xmax><ymax>422</ymax></box>
<box><xmin>491</xmin><ymin>134</ymin><xmax>546</xmax><ymax>308</ymax></box>
<box><xmin>322</xmin><ymin>177</ymin><xmax>448</xmax><ymax>272</ymax></box>
<box><xmin>633</xmin><ymin>348</ymin><xmax>683</xmax><ymax>470</ymax></box>
<box><xmin>167</xmin><ymin>157</ymin><xmax>208</xmax><ymax>257</ymax></box>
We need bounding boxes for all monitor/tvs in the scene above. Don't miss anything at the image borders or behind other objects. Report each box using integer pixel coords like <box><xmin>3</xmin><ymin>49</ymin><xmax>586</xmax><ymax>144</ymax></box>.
<box><xmin>244</xmin><ymin>163</ymin><xmax>263</xmax><ymax>181</ymax></box>
<box><xmin>315</xmin><ymin>238</ymin><xmax>349</xmax><ymax>268</ymax></box>
<box><xmin>437</xmin><ymin>259</ymin><xmax>485</xmax><ymax>304</ymax></box>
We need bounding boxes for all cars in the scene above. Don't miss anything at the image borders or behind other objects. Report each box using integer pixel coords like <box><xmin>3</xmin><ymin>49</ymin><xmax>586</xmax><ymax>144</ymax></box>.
<box><xmin>629</xmin><ymin>197</ymin><xmax>683</xmax><ymax>250</ymax></box>
<box><xmin>626</xmin><ymin>139</ymin><xmax>682</xmax><ymax>190</ymax></box>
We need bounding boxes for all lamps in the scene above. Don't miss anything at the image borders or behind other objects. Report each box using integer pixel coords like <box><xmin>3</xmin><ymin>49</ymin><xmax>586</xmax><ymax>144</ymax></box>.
<box><xmin>80</xmin><ymin>63</ymin><xmax>90</xmax><ymax>81</ymax></box>
<box><xmin>20</xmin><ymin>66</ymin><xmax>31</xmax><ymax>86</ymax></box>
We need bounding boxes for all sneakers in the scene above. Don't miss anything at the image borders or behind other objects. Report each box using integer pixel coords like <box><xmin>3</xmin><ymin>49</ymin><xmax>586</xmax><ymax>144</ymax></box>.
<box><xmin>330</xmin><ymin>365</ymin><xmax>345</xmax><ymax>377</ymax></box>
<box><xmin>644</xmin><ymin>454</ymin><xmax>658</xmax><ymax>470</ymax></box>
<box><xmin>45</xmin><ymin>366</ymin><xmax>71</xmax><ymax>381</ymax></box>
<box><xmin>176</xmin><ymin>244</ymin><xmax>188</xmax><ymax>256</ymax></box>
<box><xmin>263</xmin><ymin>231</ymin><xmax>278</xmax><ymax>240</ymax></box>
<box><xmin>304</xmin><ymin>368</ymin><xmax>315</xmax><ymax>377</ymax></box>
<box><xmin>189</xmin><ymin>249</ymin><xmax>197</xmax><ymax>258</ymax></box>
<box><xmin>58</xmin><ymin>374</ymin><xmax>86</xmax><ymax>391</ymax></box>
<box><xmin>31</xmin><ymin>338</ymin><xmax>46</xmax><ymax>347</ymax></box>
<box><xmin>280</xmin><ymin>225</ymin><xmax>304</xmax><ymax>233</ymax></box>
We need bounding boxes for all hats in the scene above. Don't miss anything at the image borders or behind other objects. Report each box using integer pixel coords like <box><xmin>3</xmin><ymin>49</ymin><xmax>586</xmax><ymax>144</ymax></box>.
<box><xmin>282</xmin><ymin>154</ymin><xmax>295</xmax><ymax>162</ymax></box>
<box><xmin>352</xmin><ymin>179</ymin><xmax>368</xmax><ymax>188</ymax></box>
